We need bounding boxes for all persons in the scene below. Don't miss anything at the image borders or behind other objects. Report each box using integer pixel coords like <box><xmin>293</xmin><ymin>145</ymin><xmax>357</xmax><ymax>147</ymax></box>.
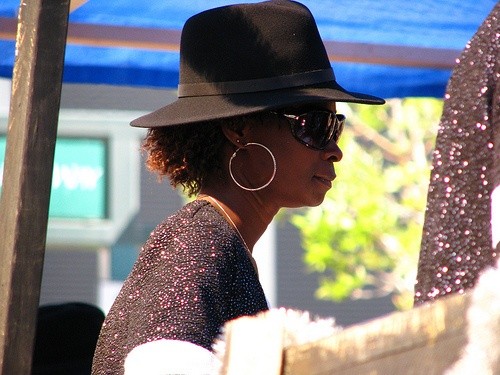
<box><xmin>412</xmin><ymin>0</ymin><xmax>499</xmax><ymax>303</ymax></box>
<box><xmin>89</xmin><ymin>0</ymin><xmax>387</xmax><ymax>375</ymax></box>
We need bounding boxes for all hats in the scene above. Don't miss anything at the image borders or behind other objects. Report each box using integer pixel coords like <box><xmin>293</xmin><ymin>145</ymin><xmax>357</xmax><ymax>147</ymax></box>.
<box><xmin>130</xmin><ymin>1</ymin><xmax>386</xmax><ymax>127</ymax></box>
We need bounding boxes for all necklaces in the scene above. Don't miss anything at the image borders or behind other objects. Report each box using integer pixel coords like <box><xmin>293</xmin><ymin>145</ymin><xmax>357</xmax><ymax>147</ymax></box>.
<box><xmin>204</xmin><ymin>193</ymin><xmax>253</xmax><ymax>255</ymax></box>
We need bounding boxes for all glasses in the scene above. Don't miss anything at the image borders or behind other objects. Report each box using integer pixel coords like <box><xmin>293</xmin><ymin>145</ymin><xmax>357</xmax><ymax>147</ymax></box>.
<box><xmin>261</xmin><ymin>107</ymin><xmax>345</xmax><ymax>151</ymax></box>
